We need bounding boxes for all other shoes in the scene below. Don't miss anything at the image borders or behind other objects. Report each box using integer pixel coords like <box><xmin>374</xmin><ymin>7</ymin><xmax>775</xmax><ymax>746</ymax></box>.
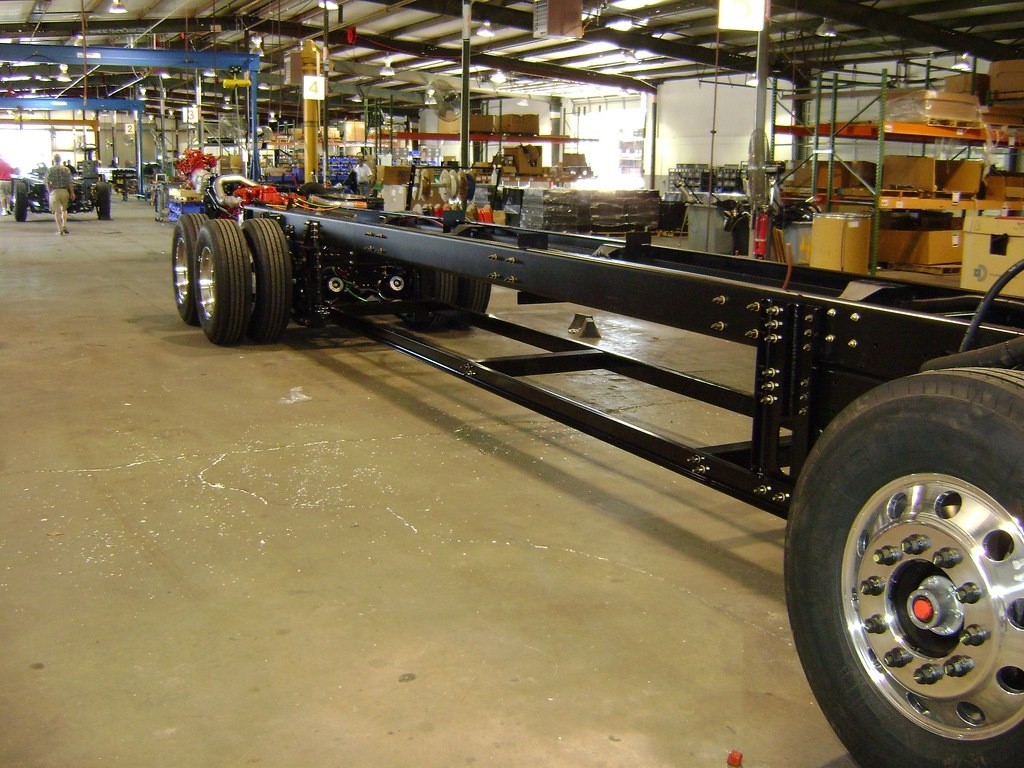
<box><xmin>63</xmin><ymin>228</ymin><xmax>69</xmax><ymax>234</ymax></box>
<box><xmin>56</xmin><ymin>231</ymin><xmax>62</xmax><ymax>237</ymax></box>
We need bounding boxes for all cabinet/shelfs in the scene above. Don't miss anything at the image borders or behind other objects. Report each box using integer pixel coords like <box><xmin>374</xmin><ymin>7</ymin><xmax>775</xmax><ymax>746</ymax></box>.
<box><xmin>809</xmin><ymin>212</ymin><xmax>871</xmax><ymax>275</ymax></box>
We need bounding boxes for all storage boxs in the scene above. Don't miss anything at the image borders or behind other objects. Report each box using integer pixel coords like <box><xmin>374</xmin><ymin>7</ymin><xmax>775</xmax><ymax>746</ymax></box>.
<box><xmin>886</xmin><ymin>90</ymin><xmax>984</xmax><ymax>129</ymax></box>
<box><xmin>784</xmin><ymin>160</ymin><xmax>853</xmax><ymax>189</ymax></box>
<box><xmin>851</xmin><ymin>161</ymin><xmax>877</xmax><ymax>187</ymax></box>
<box><xmin>441</xmin><ymin>156</ymin><xmax>459</xmax><ymax>166</ymax></box>
<box><xmin>499</xmin><ymin>146</ymin><xmax>543</xmax><ymax>181</ymax></box>
<box><xmin>471</xmin><ymin>114</ymin><xmax>494</xmax><ymax>134</ymax></box>
<box><xmin>221</xmin><ymin>155</ymin><xmax>241</xmax><ymax>167</ymax></box>
<box><xmin>294</xmin><ymin>152</ymin><xmax>306</xmax><ymax>160</ymax></box>
<box><xmin>339</xmin><ymin>121</ymin><xmax>366</xmax><ymax>142</ymax></box>
<box><xmin>267</xmin><ymin>168</ymin><xmax>286</xmax><ymax>176</ymax></box>
<box><xmin>438</xmin><ymin>112</ymin><xmax>461</xmax><ymax>135</ymax></box>
<box><xmin>288</xmin><ymin>128</ymin><xmax>302</xmax><ymax>140</ymax></box>
<box><xmin>474</xmin><ymin>161</ymin><xmax>490</xmax><ymax>175</ymax></box>
<box><xmin>563</xmin><ymin>154</ymin><xmax>586</xmax><ymax>166</ymax></box>
<box><xmin>543</xmin><ymin>167</ymin><xmax>574</xmax><ymax>175</ymax></box>
<box><xmin>883</xmin><ymin>155</ymin><xmax>982</xmax><ymax>195</ymax></box>
<box><xmin>495</xmin><ymin>114</ymin><xmax>540</xmax><ymax>136</ymax></box>
<box><xmin>944</xmin><ymin>73</ymin><xmax>990</xmax><ymax>105</ymax></box>
<box><xmin>321</xmin><ymin>127</ymin><xmax>340</xmax><ymax>140</ymax></box>
<box><xmin>876</xmin><ymin>229</ymin><xmax>963</xmax><ymax>265</ymax></box>
<box><xmin>982</xmin><ymin>176</ymin><xmax>1024</xmax><ymax>200</ymax></box>
<box><xmin>377</xmin><ymin>165</ymin><xmax>410</xmax><ymax>184</ymax></box>
<box><xmin>223</xmin><ymin>167</ymin><xmax>244</xmax><ymax>176</ymax></box>
<box><xmin>960</xmin><ymin>215</ymin><xmax>1024</xmax><ymax>297</ymax></box>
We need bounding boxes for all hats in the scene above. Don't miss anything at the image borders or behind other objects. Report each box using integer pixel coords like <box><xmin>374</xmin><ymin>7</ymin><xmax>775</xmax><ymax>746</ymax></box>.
<box><xmin>52</xmin><ymin>153</ymin><xmax>61</xmax><ymax>162</ymax></box>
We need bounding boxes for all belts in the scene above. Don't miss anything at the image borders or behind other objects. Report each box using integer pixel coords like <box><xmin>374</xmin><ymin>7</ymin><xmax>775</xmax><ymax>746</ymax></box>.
<box><xmin>53</xmin><ymin>187</ymin><xmax>67</xmax><ymax>189</ymax></box>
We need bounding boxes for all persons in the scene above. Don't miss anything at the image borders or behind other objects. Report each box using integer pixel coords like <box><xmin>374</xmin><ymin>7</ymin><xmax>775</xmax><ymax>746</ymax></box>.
<box><xmin>0</xmin><ymin>152</ymin><xmax>154</xmax><ymax>236</ymax></box>
<box><xmin>350</xmin><ymin>158</ymin><xmax>373</xmax><ymax>196</ymax></box>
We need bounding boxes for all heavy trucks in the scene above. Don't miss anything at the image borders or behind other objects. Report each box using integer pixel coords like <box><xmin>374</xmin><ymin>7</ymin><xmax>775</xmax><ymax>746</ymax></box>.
<box><xmin>10</xmin><ymin>143</ymin><xmax>111</xmax><ymax>222</ymax></box>
<box><xmin>168</xmin><ymin>179</ymin><xmax>1023</xmax><ymax>767</ymax></box>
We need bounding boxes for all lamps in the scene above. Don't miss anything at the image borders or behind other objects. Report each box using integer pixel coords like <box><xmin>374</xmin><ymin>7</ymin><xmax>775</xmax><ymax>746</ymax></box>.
<box><xmin>518</xmin><ymin>99</ymin><xmax>529</xmax><ymax>107</ymax></box>
<box><xmin>476</xmin><ymin>20</ymin><xmax>495</xmax><ymax>38</ymax></box>
<box><xmin>352</xmin><ymin>93</ymin><xmax>362</xmax><ymax>102</ymax></box>
<box><xmin>269</xmin><ymin>111</ymin><xmax>277</xmax><ymax>123</ymax></box>
<box><xmin>137</xmin><ymin>89</ymin><xmax>148</xmax><ymax>101</ymax></box>
<box><xmin>108</xmin><ymin>0</ymin><xmax>128</xmax><ymax>14</ymax></box>
<box><xmin>202</xmin><ymin>69</ymin><xmax>217</xmax><ymax>76</ymax></box>
<box><xmin>490</xmin><ymin>70</ymin><xmax>506</xmax><ymax>83</ymax></box>
<box><xmin>379</xmin><ymin>52</ymin><xmax>395</xmax><ymax>75</ymax></box>
<box><xmin>222</xmin><ymin>96</ymin><xmax>233</xmax><ymax>110</ymax></box>
<box><xmin>815</xmin><ymin>17</ymin><xmax>837</xmax><ymax>37</ymax></box>
<box><xmin>424</xmin><ymin>90</ymin><xmax>437</xmax><ymax>105</ymax></box>
<box><xmin>258</xmin><ymin>82</ymin><xmax>269</xmax><ymax>91</ymax></box>
<box><xmin>249</xmin><ymin>35</ymin><xmax>264</xmax><ymax>57</ymax></box>
<box><xmin>56</xmin><ymin>64</ymin><xmax>73</xmax><ymax>82</ymax></box>
